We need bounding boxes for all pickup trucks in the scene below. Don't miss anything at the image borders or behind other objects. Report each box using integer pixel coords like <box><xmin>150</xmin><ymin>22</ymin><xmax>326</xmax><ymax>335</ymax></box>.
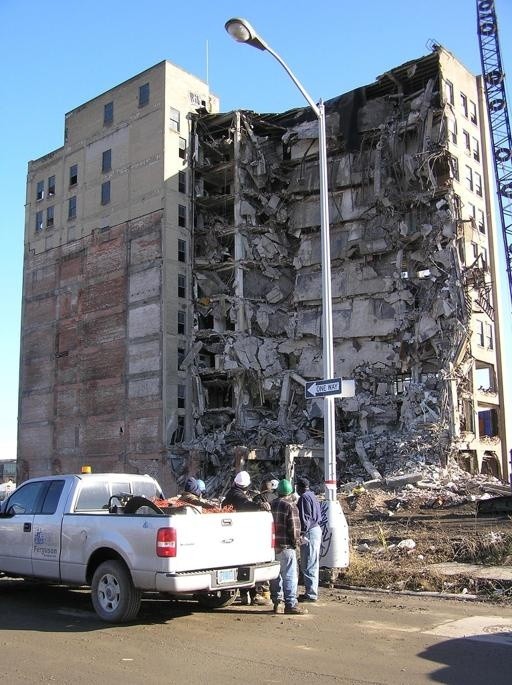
<box><xmin>0</xmin><ymin>465</ymin><xmax>283</xmax><ymax>624</ymax></box>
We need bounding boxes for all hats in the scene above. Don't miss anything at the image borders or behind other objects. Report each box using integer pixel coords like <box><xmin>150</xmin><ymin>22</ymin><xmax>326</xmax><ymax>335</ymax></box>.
<box><xmin>185</xmin><ymin>477</ymin><xmax>205</xmax><ymax>497</ymax></box>
<box><xmin>279</xmin><ymin>479</ymin><xmax>293</xmax><ymax>496</ymax></box>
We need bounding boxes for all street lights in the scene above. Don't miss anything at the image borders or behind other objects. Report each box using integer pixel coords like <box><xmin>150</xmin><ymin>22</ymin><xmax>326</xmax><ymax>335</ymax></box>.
<box><xmin>224</xmin><ymin>16</ymin><xmax>341</xmax><ymax>502</ymax></box>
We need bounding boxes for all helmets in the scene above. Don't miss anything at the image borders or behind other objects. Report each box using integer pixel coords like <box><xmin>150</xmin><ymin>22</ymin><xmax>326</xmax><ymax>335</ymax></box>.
<box><xmin>234</xmin><ymin>471</ymin><xmax>250</xmax><ymax>485</ymax></box>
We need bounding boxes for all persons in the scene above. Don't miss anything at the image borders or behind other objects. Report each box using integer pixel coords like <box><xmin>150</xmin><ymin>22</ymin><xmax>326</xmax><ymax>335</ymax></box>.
<box><xmin>196</xmin><ymin>479</ymin><xmax>206</xmax><ymax>495</ymax></box>
<box><xmin>176</xmin><ymin>475</ymin><xmax>219</xmax><ymax>508</ymax></box>
<box><xmin>223</xmin><ymin>470</ymin><xmax>272</xmax><ymax>605</ymax></box>
<box><xmin>268</xmin><ymin>477</ymin><xmax>310</xmax><ymax>615</ymax></box>
<box><xmin>251</xmin><ymin>476</ymin><xmax>323</xmax><ymax>603</ymax></box>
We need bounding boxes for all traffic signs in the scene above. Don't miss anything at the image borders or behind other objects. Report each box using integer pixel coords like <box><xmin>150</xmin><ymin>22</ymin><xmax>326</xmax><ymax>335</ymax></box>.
<box><xmin>304</xmin><ymin>378</ymin><xmax>341</xmax><ymax>398</ymax></box>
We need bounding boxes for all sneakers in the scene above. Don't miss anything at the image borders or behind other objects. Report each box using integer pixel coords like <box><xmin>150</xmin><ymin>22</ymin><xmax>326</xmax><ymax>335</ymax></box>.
<box><xmin>241</xmin><ymin>592</ymin><xmax>316</xmax><ymax>614</ymax></box>
